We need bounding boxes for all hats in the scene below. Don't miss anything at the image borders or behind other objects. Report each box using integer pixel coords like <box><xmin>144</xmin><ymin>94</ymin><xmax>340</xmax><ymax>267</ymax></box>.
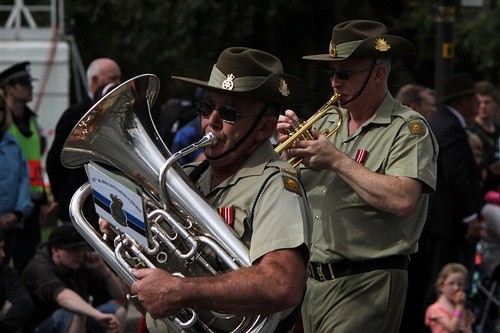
<box><xmin>435</xmin><ymin>73</ymin><xmax>479</xmax><ymax>106</ymax></box>
<box><xmin>302</xmin><ymin>20</ymin><xmax>415</xmax><ymax>62</ymax></box>
<box><xmin>0</xmin><ymin>61</ymin><xmax>37</xmax><ymax>89</ymax></box>
<box><xmin>171</xmin><ymin>47</ymin><xmax>313</xmax><ymax>107</ymax></box>
<box><xmin>48</xmin><ymin>226</ymin><xmax>88</xmax><ymax>249</ymax></box>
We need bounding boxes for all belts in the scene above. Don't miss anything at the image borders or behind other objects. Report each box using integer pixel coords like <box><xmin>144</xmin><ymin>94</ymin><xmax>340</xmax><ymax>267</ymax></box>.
<box><xmin>309</xmin><ymin>254</ymin><xmax>409</xmax><ymax>282</ymax></box>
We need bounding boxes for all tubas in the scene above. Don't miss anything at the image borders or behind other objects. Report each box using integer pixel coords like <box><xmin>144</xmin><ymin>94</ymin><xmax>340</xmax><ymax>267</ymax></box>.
<box><xmin>60</xmin><ymin>74</ymin><xmax>284</xmax><ymax>333</ymax></box>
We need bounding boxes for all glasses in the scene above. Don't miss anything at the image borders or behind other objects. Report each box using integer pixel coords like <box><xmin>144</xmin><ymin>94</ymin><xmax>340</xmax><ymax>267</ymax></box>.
<box><xmin>195</xmin><ymin>99</ymin><xmax>271</xmax><ymax>124</ymax></box>
<box><xmin>323</xmin><ymin>67</ymin><xmax>374</xmax><ymax>80</ymax></box>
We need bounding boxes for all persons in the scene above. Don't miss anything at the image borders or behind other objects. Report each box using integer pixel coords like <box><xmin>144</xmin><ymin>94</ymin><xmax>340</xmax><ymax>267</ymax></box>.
<box><xmin>23</xmin><ymin>224</ymin><xmax>125</xmax><ymax>333</ymax></box>
<box><xmin>425</xmin><ymin>264</ymin><xmax>474</xmax><ymax>333</ymax></box>
<box><xmin>98</xmin><ymin>49</ymin><xmax>315</xmax><ymax>333</ymax></box>
<box><xmin>46</xmin><ymin>59</ymin><xmax>120</xmax><ymax>236</ymax></box>
<box><xmin>276</xmin><ymin>20</ymin><xmax>438</xmax><ymax>333</ymax></box>
<box><xmin>397</xmin><ymin>81</ymin><xmax>500</xmax><ymax>333</ymax></box>
<box><xmin>0</xmin><ymin>232</ymin><xmax>31</xmax><ymax>333</ymax></box>
<box><xmin>0</xmin><ymin>62</ymin><xmax>57</xmax><ymax>277</ymax></box>
<box><xmin>0</xmin><ymin>92</ymin><xmax>34</xmax><ymax>256</ymax></box>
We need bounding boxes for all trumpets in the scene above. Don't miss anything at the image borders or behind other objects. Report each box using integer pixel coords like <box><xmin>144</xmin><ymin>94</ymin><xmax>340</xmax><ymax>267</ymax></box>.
<box><xmin>274</xmin><ymin>93</ymin><xmax>343</xmax><ymax>169</ymax></box>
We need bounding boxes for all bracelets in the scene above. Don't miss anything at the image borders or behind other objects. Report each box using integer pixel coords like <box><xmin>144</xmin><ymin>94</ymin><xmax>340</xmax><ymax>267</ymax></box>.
<box><xmin>103</xmin><ymin>271</ymin><xmax>112</xmax><ymax>281</ymax></box>
<box><xmin>453</xmin><ymin>310</ymin><xmax>463</xmax><ymax>318</ymax></box>
